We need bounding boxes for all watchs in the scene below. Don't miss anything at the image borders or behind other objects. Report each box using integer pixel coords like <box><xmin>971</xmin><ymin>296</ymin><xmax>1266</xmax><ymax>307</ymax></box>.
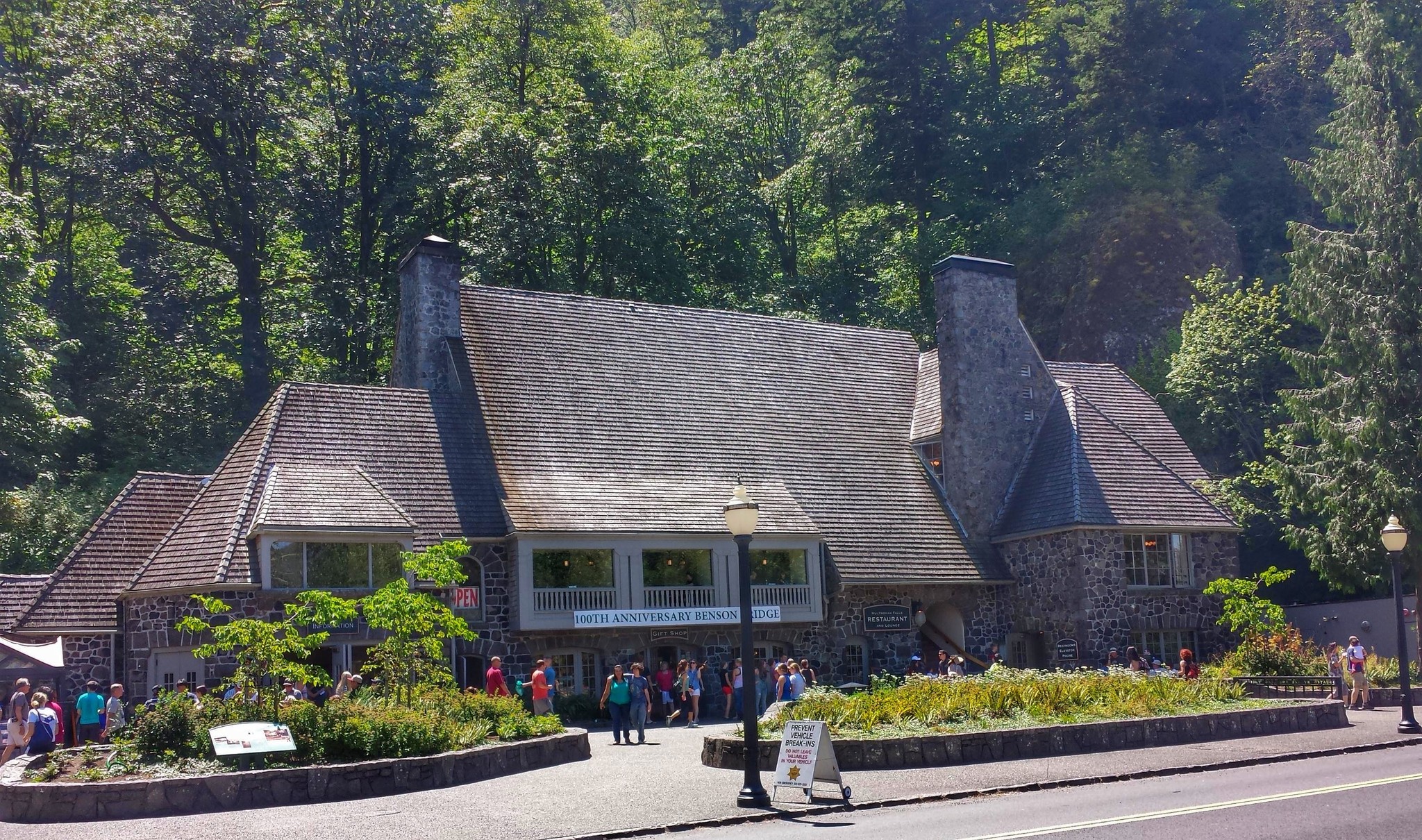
<box><xmin>19</xmin><ymin>723</ymin><xmax>24</xmax><ymax>726</ymax></box>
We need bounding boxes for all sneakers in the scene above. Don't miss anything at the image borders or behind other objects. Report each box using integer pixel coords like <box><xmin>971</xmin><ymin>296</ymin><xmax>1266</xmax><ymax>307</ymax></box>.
<box><xmin>612</xmin><ymin>715</ymin><xmax>740</xmax><ymax>745</ymax></box>
<box><xmin>1343</xmin><ymin>702</ymin><xmax>1374</xmax><ymax>710</ymax></box>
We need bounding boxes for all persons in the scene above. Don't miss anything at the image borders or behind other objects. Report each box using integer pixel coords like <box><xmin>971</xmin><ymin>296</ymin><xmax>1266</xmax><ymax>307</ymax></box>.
<box><xmin>902</xmin><ymin>632</ymin><xmax>1381</xmax><ymax>713</ymax></box>
<box><xmin>74</xmin><ymin>681</ymin><xmax>107</xmax><ymax>747</ymax></box>
<box><xmin>23</xmin><ymin>692</ymin><xmax>59</xmax><ymax>754</ymax></box>
<box><xmin>101</xmin><ymin>668</ymin><xmax>483</xmax><ymax>744</ymax></box>
<box><xmin>600</xmin><ymin>665</ymin><xmax>634</xmax><ymax>744</ymax></box>
<box><xmin>37</xmin><ymin>686</ymin><xmax>66</xmax><ymax>751</ymax></box>
<box><xmin>326</xmin><ymin>545</ymin><xmax>350</xmax><ymax>589</ymax></box>
<box><xmin>521</xmin><ymin>656</ymin><xmax>554</xmax><ymax>703</ymax></box>
<box><xmin>532</xmin><ymin>659</ymin><xmax>554</xmax><ymax>719</ymax></box>
<box><xmin>0</xmin><ymin>678</ymin><xmax>30</xmax><ymax>766</ymax></box>
<box><xmin>636</xmin><ymin>656</ymin><xmax>831</xmax><ymax>728</ymax></box>
<box><xmin>485</xmin><ymin>656</ymin><xmax>513</xmax><ymax>700</ymax></box>
<box><xmin>607</xmin><ymin>662</ymin><xmax>652</xmax><ymax>744</ymax></box>
<box><xmin>679</xmin><ymin>568</ymin><xmax>793</xmax><ymax>607</ymax></box>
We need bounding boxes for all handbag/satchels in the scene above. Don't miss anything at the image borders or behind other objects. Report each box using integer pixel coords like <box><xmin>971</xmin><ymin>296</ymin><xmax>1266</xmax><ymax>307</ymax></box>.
<box><xmin>28</xmin><ymin>708</ymin><xmax>53</xmax><ymax>751</ymax></box>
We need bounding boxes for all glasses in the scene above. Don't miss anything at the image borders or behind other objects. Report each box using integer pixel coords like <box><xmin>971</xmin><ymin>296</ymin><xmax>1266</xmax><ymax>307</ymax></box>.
<box><xmin>690</xmin><ymin>662</ymin><xmax>695</xmax><ymax>664</ymax></box>
<box><xmin>938</xmin><ymin>654</ymin><xmax>942</xmax><ymax>655</ymax></box>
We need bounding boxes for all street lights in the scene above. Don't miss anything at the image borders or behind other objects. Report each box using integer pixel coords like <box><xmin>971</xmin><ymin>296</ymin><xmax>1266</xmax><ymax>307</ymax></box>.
<box><xmin>1380</xmin><ymin>506</ymin><xmax>1422</xmax><ymax>734</ymax></box>
<box><xmin>723</xmin><ymin>473</ymin><xmax>772</xmax><ymax>807</ymax></box>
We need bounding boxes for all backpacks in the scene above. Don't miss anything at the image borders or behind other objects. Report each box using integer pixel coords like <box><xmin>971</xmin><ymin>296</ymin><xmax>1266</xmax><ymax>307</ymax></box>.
<box><xmin>672</xmin><ymin>672</ymin><xmax>686</xmax><ymax>692</ymax></box>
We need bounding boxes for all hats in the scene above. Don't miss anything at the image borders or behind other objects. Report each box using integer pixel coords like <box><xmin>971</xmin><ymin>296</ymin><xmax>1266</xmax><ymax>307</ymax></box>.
<box><xmin>774</xmin><ymin>663</ymin><xmax>787</xmax><ymax>671</ymax></box>
<box><xmin>1110</xmin><ymin>647</ymin><xmax>1117</xmax><ymax>653</ymax></box>
<box><xmin>1151</xmin><ymin>660</ymin><xmax>1161</xmax><ymax>666</ymax></box>
<box><xmin>283</xmin><ymin>678</ymin><xmax>293</xmax><ymax>685</ymax></box>
<box><xmin>152</xmin><ymin>685</ymin><xmax>162</xmax><ymax>695</ymax></box>
<box><xmin>347</xmin><ymin>674</ymin><xmax>363</xmax><ymax>684</ymax></box>
<box><xmin>370</xmin><ymin>679</ymin><xmax>383</xmax><ymax>685</ymax></box>
<box><xmin>947</xmin><ymin>654</ymin><xmax>964</xmax><ymax>664</ymax></box>
<box><xmin>911</xmin><ymin>656</ymin><xmax>921</xmax><ymax>661</ymax></box>
